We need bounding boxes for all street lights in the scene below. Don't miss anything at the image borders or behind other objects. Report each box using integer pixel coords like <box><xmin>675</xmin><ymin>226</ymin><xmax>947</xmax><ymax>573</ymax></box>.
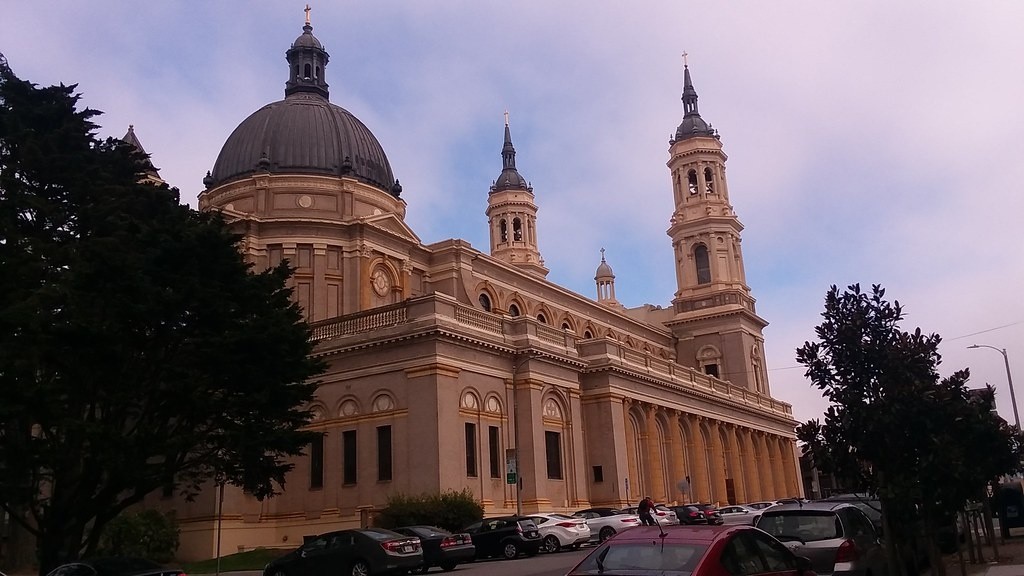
<box><xmin>966</xmin><ymin>343</ymin><xmax>1022</xmax><ymax>437</ymax></box>
<box><xmin>511</xmin><ymin>354</ymin><xmax>551</xmax><ymax>516</ymax></box>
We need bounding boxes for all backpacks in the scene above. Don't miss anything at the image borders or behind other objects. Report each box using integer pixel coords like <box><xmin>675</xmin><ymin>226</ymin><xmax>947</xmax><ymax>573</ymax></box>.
<box><xmin>637</xmin><ymin>501</ymin><xmax>650</xmax><ymax>513</ymax></box>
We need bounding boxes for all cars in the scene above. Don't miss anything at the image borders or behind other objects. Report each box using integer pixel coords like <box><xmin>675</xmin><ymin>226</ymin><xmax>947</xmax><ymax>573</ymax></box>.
<box><xmin>45</xmin><ymin>557</ymin><xmax>188</xmax><ymax>576</ymax></box>
<box><xmin>820</xmin><ymin>500</ymin><xmax>885</xmax><ymax>544</ymax></box>
<box><xmin>569</xmin><ymin>507</ymin><xmax>642</xmax><ymax>543</ymax></box>
<box><xmin>747</xmin><ymin>500</ymin><xmax>779</xmax><ymax>513</ymax></box>
<box><xmin>388</xmin><ymin>525</ymin><xmax>475</xmax><ymax>575</ymax></box>
<box><xmin>455</xmin><ymin>514</ymin><xmax>541</xmax><ymax>559</ymax></box>
<box><xmin>754</xmin><ymin>502</ymin><xmax>892</xmax><ymax>576</ymax></box>
<box><xmin>564</xmin><ymin>524</ymin><xmax>822</xmax><ymax>576</ymax></box>
<box><xmin>717</xmin><ymin>504</ymin><xmax>765</xmax><ymax>525</ymax></box>
<box><xmin>523</xmin><ymin>513</ymin><xmax>591</xmax><ymax>553</ymax></box>
<box><xmin>262</xmin><ymin>528</ymin><xmax>424</xmax><ymax>576</ymax></box>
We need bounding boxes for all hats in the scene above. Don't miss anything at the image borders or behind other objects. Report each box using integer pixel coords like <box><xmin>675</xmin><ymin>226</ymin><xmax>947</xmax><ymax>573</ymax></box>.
<box><xmin>646</xmin><ymin>496</ymin><xmax>652</xmax><ymax>499</ymax></box>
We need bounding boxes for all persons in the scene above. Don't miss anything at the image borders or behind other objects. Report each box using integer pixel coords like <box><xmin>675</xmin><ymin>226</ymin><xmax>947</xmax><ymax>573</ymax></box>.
<box><xmin>639</xmin><ymin>496</ymin><xmax>657</xmax><ymax>526</ymax></box>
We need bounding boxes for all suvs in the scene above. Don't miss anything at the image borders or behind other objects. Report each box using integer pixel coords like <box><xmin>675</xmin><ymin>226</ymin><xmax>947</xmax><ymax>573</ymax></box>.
<box><xmin>699</xmin><ymin>503</ymin><xmax>724</xmax><ymax>526</ymax></box>
<box><xmin>668</xmin><ymin>504</ymin><xmax>709</xmax><ymax>527</ymax></box>
<box><xmin>619</xmin><ymin>503</ymin><xmax>680</xmax><ymax>526</ymax></box>
